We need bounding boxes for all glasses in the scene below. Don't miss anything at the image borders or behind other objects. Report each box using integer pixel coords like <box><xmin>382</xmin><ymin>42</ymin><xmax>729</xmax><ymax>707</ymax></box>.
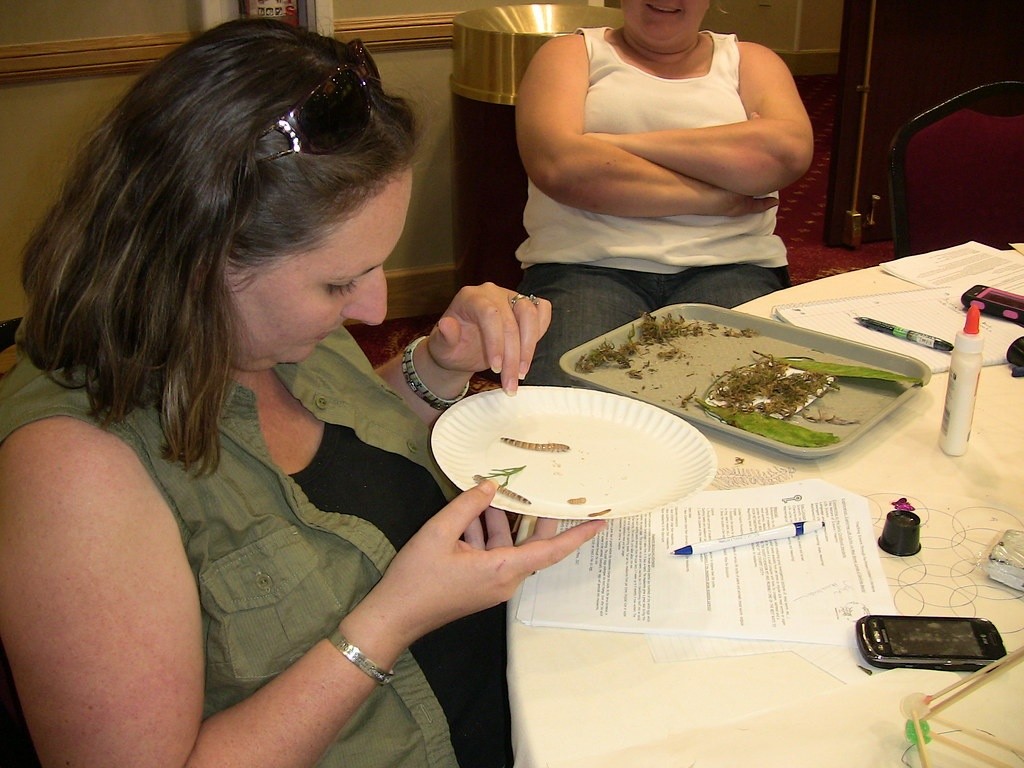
<box><xmin>255</xmin><ymin>37</ymin><xmax>383</xmax><ymax>164</ymax></box>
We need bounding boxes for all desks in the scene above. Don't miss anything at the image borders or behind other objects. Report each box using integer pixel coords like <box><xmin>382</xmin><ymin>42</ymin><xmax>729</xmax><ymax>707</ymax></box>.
<box><xmin>503</xmin><ymin>247</ymin><xmax>1024</xmax><ymax>767</ymax></box>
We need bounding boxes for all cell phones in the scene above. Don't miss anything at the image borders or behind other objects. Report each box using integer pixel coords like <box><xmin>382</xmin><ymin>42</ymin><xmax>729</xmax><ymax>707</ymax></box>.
<box><xmin>961</xmin><ymin>284</ymin><xmax>1024</xmax><ymax>325</ymax></box>
<box><xmin>857</xmin><ymin>614</ymin><xmax>1008</xmax><ymax>673</ymax></box>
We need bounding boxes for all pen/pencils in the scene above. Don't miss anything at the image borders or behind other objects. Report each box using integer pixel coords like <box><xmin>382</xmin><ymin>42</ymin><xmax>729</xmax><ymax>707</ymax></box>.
<box><xmin>854</xmin><ymin>316</ymin><xmax>954</xmax><ymax>352</ymax></box>
<box><xmin>669</xmin><ymin>520</ymin><xmax>826</xmax><ymax>556</ymax></box>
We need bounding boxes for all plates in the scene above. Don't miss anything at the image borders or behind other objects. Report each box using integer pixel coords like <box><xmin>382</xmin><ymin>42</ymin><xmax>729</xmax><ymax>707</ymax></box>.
<box><xmin>430</xmin><ymin>385</ymin><xmax>717</xmax><ymax>520</ymax></box>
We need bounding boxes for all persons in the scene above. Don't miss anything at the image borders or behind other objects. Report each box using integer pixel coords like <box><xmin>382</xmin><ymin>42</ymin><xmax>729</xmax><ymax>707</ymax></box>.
<box><xmin>515</xmin><ymin>0</ymin><xmax>813</xmax><ymax>388</ymax></box>
<box><xmin>0</xmin><ymin>20</ymin><xmax>607</xmax><ymax>768</ymax></box>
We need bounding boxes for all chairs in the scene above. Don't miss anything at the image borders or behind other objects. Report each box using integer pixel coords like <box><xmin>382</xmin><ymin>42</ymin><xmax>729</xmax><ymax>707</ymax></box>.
<box><xmin>891</xmin><ymin>79</ymin><xmax>1024</xmax><ymax>256</ymax></box>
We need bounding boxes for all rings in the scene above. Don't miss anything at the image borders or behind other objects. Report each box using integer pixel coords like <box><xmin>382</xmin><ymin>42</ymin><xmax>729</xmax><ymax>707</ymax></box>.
<box><xmin>510</xmin><ymin>291</ymin><xmax>539</xmax><ymax>309</ymax></box>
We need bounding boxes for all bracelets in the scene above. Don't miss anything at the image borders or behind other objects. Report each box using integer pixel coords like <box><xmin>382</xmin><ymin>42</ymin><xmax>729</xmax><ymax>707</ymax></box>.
<box><xmin>328</xmin><ymin>629</ymin><xmax>394</xmax><ymax>685</ymax></box>
<box><xmin>402</xmin><ymin>336</ymin><xmax>469</xmax><ymax>409</ymax></box>
